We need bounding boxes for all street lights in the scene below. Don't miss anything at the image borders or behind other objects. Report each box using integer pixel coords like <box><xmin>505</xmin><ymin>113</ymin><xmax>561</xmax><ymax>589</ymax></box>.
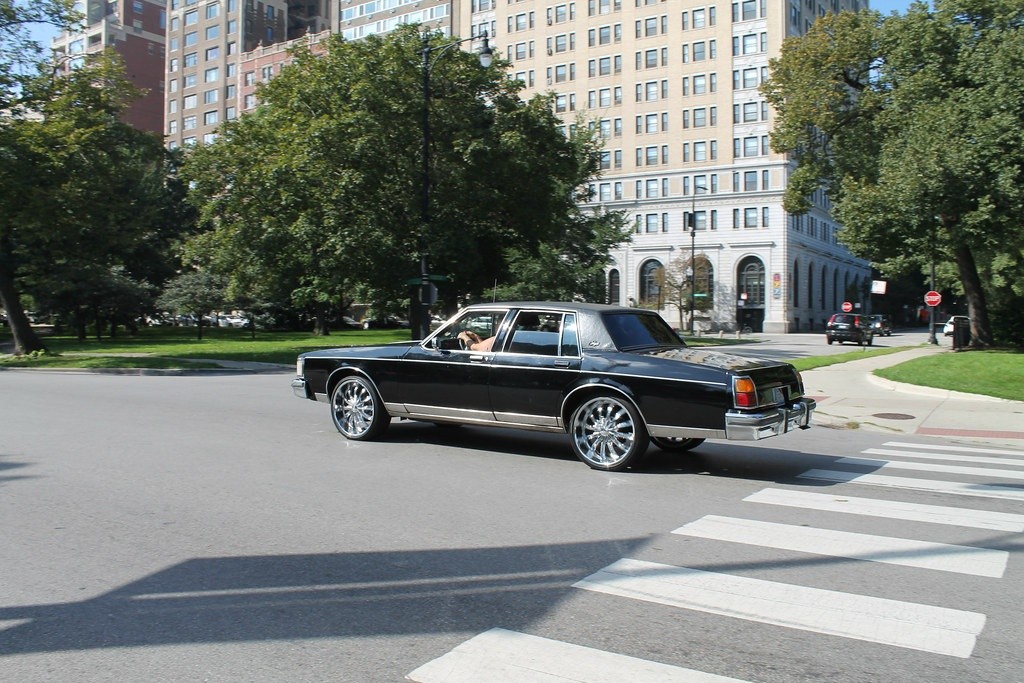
<box><xmin>690</xmin><ymin>186</ymin><xmax>709</xmax><ymax>335</ymax></box>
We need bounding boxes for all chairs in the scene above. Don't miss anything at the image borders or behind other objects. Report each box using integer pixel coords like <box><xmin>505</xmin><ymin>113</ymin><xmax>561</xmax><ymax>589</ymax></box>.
<box><xmin>518</xmin><ymin>313</ymin><xmax>540</xmax><ymax>331</ymax></box>
<box><xmin>541</xmin><ymin>314</ymin><xmax>559</xmax><ymax>332</ymax></box>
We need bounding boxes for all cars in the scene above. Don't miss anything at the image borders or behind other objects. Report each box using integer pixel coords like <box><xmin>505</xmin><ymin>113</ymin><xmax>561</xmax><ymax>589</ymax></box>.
<box><xmin>0</xmin><ymin>309</ymin><xmax>40</xmax><ymax>324</ymax></box>
<box><xmin>340</xmin><ymin>313</ymin><xmax>410</xmax><ymax>331</ymax></box>
<box><xmin>133</xmin><ymin>311</ymin><xmax>252</xmax><ymax>329</ymax></box>
<box><xmin>867</xmin><ymin>314</ymin><xmax>892</xmax><ymax>338</ymax></box>
<box><xmin>291</xmin><ymin>300</ymin><xmax>816</xmax><ymax>473</ymax></box>
<box><xmin>943</xmin><ymin>315</ymin><xmax>970</xmax><ymax>335</ymax></box>
<box><xmin>429</xmin><ymin>313</ymin><xmax>493</xmax><ymax>331</ymax></box>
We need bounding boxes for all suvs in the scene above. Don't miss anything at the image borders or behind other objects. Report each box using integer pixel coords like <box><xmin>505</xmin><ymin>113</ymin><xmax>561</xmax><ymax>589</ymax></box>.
<box><xmin>825</xmin><ymin>313</ymin><xmax>873</xmax><ymax>346</ymax></box>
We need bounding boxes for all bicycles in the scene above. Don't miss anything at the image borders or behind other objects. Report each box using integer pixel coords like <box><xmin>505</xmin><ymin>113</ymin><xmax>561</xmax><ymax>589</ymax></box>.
<box><xmin>735</xmin><ymin>322</ymin><xmax>752</xmax><ymax>335</ymax></box>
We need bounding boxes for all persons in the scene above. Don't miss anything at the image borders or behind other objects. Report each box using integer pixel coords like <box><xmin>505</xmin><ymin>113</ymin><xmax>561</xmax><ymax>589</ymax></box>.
<box><xmin>908</xmin><ymin>306</ymin><xmax>929</xmax><ymax>328</ymax></box>
<box><xmin>457</xmin><ymin>314</ymin><xmax>505</xmax><ymax>351</ymax></box>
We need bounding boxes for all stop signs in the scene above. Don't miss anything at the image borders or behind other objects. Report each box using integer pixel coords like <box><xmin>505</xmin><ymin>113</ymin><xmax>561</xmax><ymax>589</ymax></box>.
<box><xmin>841</xmin><ymin>300</ymin><xmax>853</xmax><ymax>312</ymax></box>
<box><xmin>923</xmin><ymin>290</ymin><xmax>941</xmax><ymax>306</ymax></box>
